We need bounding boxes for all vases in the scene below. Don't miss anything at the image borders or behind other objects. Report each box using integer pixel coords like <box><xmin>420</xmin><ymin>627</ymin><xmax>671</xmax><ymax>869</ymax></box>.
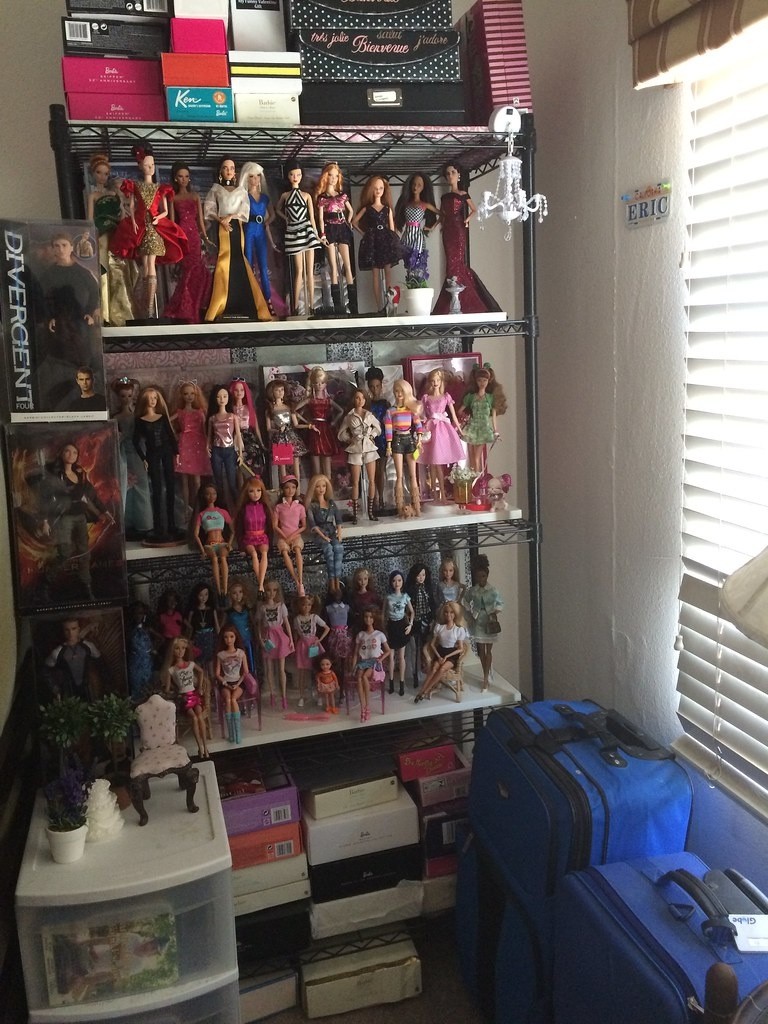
<box><xmin>44</xmin><ymin>818</ymin><xmax>90</xmax><ymax>865</ymax></box>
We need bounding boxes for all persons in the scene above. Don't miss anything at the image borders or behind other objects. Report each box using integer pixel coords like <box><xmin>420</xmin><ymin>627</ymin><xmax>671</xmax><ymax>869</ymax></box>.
<box><xmin>64</xmin><ymin>932</ymin><xmax>169</xmax><ymax>993</ymax></box>
<box><xmin>30</xmin><ymin>143</ymin><xmax>506</xmax><ymax>778</ymax></box>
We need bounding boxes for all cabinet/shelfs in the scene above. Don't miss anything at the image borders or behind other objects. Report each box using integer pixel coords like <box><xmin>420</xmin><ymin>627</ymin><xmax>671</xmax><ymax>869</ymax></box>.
<box><xmin>13</xmin><ymin>759</ymin><xmax>242</xmax><ymax>1024</ymax></box>
<box><xmin>47</xmin><ymin>101</ymin><xmax>545</xmax><ymax>983</ymax></box>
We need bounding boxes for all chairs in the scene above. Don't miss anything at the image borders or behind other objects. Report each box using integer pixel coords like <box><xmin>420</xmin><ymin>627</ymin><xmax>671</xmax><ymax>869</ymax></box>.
<box><xmin>127</xmin><ymin>690</ymin><xmax>200</xmax><ymax>827</ymax></box>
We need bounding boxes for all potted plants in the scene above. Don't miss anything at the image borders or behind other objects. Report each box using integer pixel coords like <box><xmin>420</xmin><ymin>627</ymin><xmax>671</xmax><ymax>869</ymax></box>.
<box><xmin>39</xmin><ymin>692</ymin><xmax>139</xmax><ymax>811</ymax></box>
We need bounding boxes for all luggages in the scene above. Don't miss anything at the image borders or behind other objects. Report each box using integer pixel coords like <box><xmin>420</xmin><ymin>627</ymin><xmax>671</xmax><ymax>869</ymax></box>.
<box><xmin>452</xmin><ymin>698</ymin><xmax>768</xmax><ymax>1024</ymax></box>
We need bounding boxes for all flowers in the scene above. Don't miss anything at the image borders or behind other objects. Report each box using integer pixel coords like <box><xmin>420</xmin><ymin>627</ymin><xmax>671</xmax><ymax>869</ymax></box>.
<box><xmin>43</xmin><ymin>765</ymin><xmax>87</xmax><ymax>828</ymax></box>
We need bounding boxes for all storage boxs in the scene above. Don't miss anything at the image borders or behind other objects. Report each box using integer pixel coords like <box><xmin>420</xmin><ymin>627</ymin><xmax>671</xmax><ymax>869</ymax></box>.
<box><xmin>210</xmin><ymin>716</ymin><xmax>472</xmax><ymax>1024</ymax></box>
<box><xmin>60</xmin><ymin>0</ymin><xmax>533</xmax><ymax>127</ymax></box>
<box><xmin>0</xmin><ymin>218</ymin><xmax>132</xmax><ymax>698</ymax></box>
